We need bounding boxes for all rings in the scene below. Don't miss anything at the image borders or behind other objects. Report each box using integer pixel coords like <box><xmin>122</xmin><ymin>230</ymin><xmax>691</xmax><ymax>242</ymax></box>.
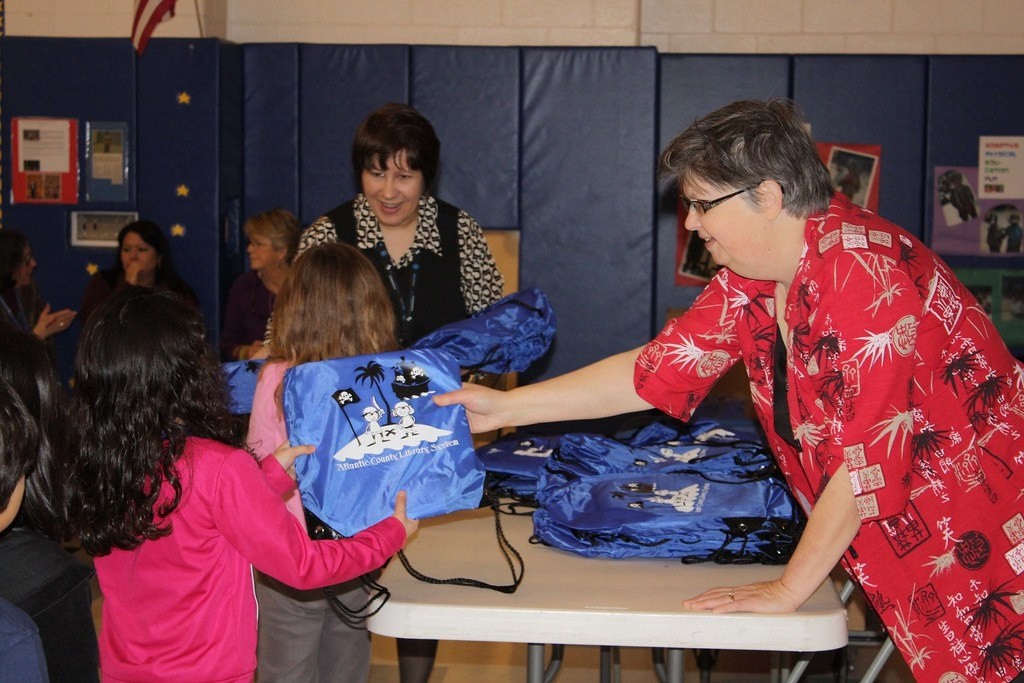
<box><xmin>727</xmin><ymin>592</ymin><xmax>735</xmax><ymax>604</ymax></box>
<box><xmin>59</xmin><ymin>321</ymin><xmax>65</xmax><ymax>328</ymax></box>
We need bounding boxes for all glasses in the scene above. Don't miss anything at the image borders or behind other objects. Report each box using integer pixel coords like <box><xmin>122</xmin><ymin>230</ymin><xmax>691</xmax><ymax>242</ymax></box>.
<box><xmin>678</xmin><ymin>179</ymin><xmax>785</xmax><ymax>219</ymax></box>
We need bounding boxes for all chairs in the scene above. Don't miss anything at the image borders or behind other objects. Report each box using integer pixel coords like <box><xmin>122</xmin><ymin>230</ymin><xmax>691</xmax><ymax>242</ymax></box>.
<box><xmin>787</xmin><ymin>581</ymin><xmax>893</xmax><ymax>683</ymax></box>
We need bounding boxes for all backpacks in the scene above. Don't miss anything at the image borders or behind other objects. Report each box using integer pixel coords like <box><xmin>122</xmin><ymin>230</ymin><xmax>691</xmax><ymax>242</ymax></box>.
<box><xmin>282</xmin><ymin>288</ymin><xmax>811</xmax><ymax>558</ymax></box>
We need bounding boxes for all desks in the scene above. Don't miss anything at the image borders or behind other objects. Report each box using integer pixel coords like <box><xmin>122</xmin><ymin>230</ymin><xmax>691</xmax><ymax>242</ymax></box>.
<box><xmin>368</xmin><ymin>499</ymin><xmax>848</xmax><ymax>683</ymax></box>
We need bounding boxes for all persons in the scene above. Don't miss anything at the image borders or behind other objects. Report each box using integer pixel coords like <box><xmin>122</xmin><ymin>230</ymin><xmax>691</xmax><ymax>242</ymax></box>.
<box><xmin>76</xmin><ymin>219</ymin><xmax>196</xmax><ymax>331</ymax></box>
<box><xmin>246</xmin><ymin>244</ymin><xmax>400</xmax><ymax>683</ymax></box>
<box><xmin>985</xmin><ymin>213</ymin><xmax>1022</xmax><ymax>252</ymax></box>
<box><xmin>0</xmin><ymin>225</ymin><xmax>103</xmax><ymax>683</ymax></box>
<box><xmin>218</xmin><ymin>207</ymin><xmax>306</xmax><ymax>363</ymax></box>
<box><xmin>265</xmin><ymin>102</ymin><xmax>505</xmax><ymax>683</ymax></box>
<box><xmin>55</xmin><ymin>290</ymin><xmax>420</xmax><ymax>683</ymax></box>
<box><xmin>837</xmin><ymin>159</ymin><xmax>861</xmax><ymax>199</ymax></box>
<box><xmin>430</xmin><ymin>96</ymin><xmax>1024</xmax><ymax>682</ymax></box>
<box><xmin>941</xmin><ymin>173</ymin><xmax>978</xmax><ymax>221</ymax></box>
